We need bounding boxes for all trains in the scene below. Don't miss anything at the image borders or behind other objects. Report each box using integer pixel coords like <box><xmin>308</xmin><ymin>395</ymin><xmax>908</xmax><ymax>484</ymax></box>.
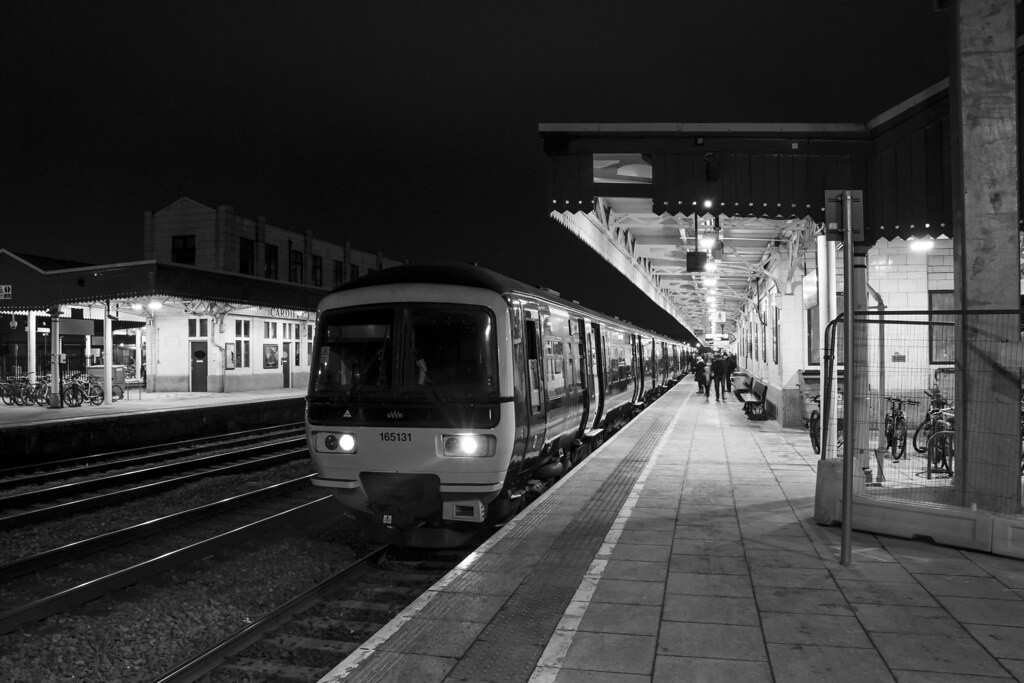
<box><xmin>305</xmin><ymin>262</ymin><xmax>698</xmax><ymax>544</ymax></box>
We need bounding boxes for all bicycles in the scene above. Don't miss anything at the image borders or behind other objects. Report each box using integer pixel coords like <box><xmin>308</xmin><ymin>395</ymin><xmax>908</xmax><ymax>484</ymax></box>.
<box><xmin>913</xmin><ymin>391</ymin><xmax>954</xmax><ymax>472</ymax></box>
<box><xmin>884</xmin><ymin>396</ymin><xmax>922</xmax><ymax>459</ymax></box>
<box><xmin>0</xmin><ymin>372</ymin><xmax>124</xmax><ymax>408</ymax></box>
<box><xmin>805</xmin><ymin>387</ymin><xmax>845</xmax><ymax>454</ymax></box>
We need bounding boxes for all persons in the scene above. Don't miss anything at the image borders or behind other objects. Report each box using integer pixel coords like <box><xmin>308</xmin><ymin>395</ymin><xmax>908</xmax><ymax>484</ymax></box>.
<box><xmin>696</xmin><ymin>348</ymin><xmax>735</xmax><ymax>402</ymax></box>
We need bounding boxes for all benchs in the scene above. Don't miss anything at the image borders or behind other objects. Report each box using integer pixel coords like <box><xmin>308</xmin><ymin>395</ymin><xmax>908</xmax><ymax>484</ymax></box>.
<box><xmin>733</xmin><ymin>373</ymin><xmax>754</xmax><ymax>401</ymax></box>
<box><xmin>740</xmin><ymin>380</ymin><xmax>768</xmax><ymax>420</ymax></box>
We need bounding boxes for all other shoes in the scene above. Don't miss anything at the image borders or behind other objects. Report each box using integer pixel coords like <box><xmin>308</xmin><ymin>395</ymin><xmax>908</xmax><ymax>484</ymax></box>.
<box><xmin>715</xmin><ymin>398</ymin><xmax>718</xmax><ymax>401</ymax></box>
<box><xmin>696</xmin><ymin>389</ymin><xmax>702</xmax><ymax>393</ymax></box>
<box><xmin>706</xmin><ymin>399</ymin><xmax>708</xmax><ymax>400</ymax></box>
<box><xmin>722</xmin><ymin>398</ymin><xmax>726</xmax><ymax>399</ymax></box>
<box><xmin>725</xmin><ymin>389</ymin><xmax>729</xmax><ymax>391</ymax></box>
